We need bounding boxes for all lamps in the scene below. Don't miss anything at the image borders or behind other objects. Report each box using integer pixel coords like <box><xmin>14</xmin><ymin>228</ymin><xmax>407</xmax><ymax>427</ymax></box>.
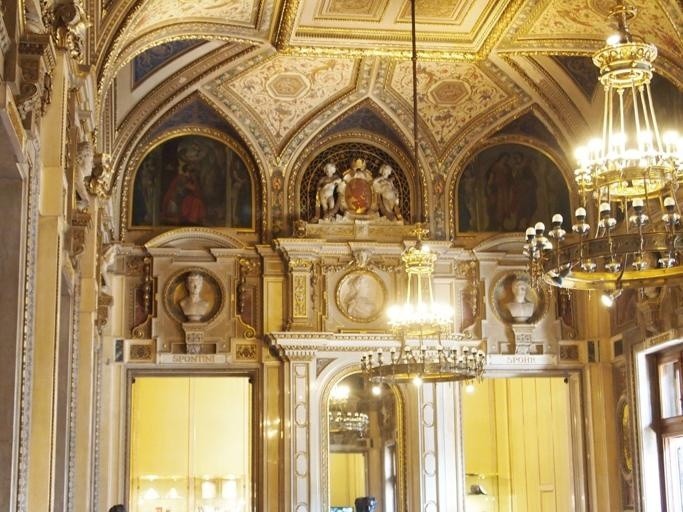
<box><xmin>523</xmin><ymin>0</ymin><xmax>683</xmax><ymax>306</ymax></box>
<box><xmin>360</xmin><ymin>0</ymin><xmax>484</xmax><ymax>396</ymax></box>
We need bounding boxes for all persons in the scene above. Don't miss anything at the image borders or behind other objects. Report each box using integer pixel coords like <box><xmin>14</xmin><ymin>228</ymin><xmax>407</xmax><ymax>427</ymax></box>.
<box><xmin>161</xmin><ymin>140</ymin><xmax>224</xmax><ymax>224</ymax></box>
<box><xmin>373</xmin><ymin>165</ymin><xmax>402</xmax><ymax>220</ymax></box>
<box><xmin>506</xmin><ymin>279</ymin><xmax>534</xmax><ymax>317</ymax></box>
<box><xmin>179</xmin><ymin>272</ymin><xmax>209</xmax><ymax>315</ymax></box>
<box><xmin>317</xmin><ymin>162</ymin><xmax>342</xmax><ymax>221</ymax></box>
<box><xmin>485</xmin><ymin>151</ymin><xmax>537</xmax><ymax>231</ymax></box>
